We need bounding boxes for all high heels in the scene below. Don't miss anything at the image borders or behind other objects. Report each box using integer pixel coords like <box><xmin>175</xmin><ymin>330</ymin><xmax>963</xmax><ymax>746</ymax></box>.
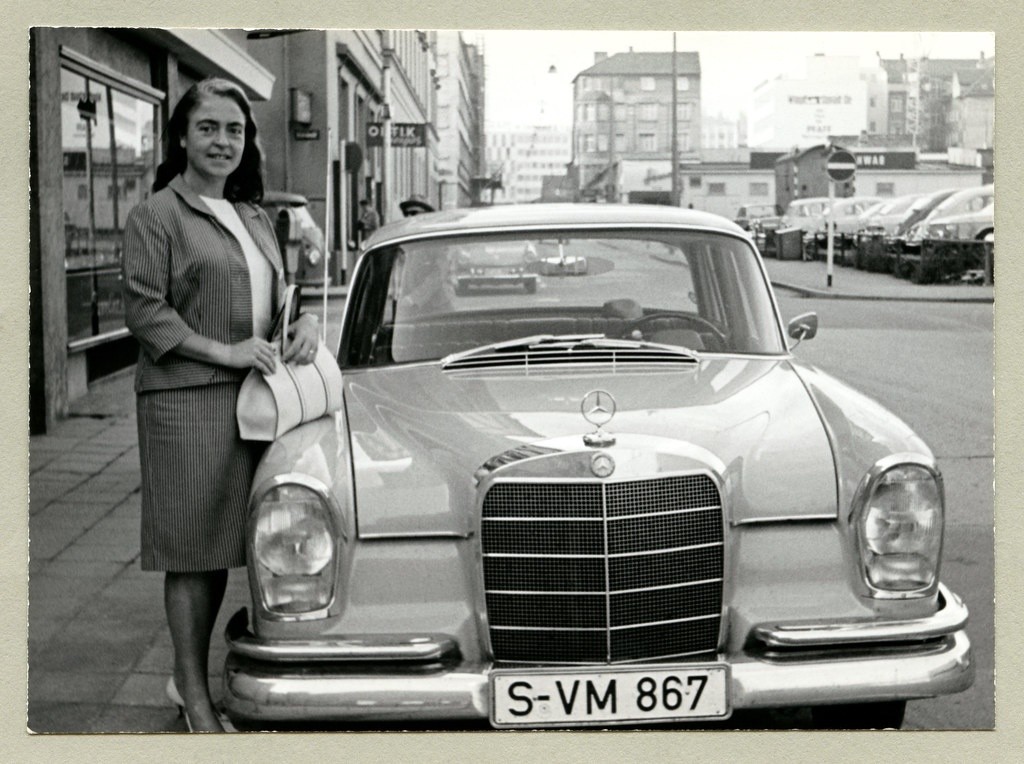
<box><xmin>183</xmin><ymin>708</ymin><xmax>217</xmax><ymax>733</ymax></box>
<box><xmin>166</xmin><ymin>676</ymin><xmax>187</xmax><ymax>714</ymax></box>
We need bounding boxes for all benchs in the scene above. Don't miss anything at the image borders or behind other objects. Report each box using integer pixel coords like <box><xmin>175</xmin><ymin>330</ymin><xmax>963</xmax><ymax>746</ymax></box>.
<box><xmin>376</xmin><ymin>318</ymin><xmax>722</xmax><ymax>364</ymax></box>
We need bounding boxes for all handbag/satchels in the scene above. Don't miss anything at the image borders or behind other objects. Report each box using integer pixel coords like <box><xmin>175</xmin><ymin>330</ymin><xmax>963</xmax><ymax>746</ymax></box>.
<box><xmin>236</xmin><ymin>282</ymin><xmax>343</xmax><ymax>442</ymax></box>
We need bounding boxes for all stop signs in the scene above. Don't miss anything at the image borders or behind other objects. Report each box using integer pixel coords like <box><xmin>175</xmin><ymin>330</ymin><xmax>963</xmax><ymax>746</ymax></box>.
<box><xmin>827</xmin><ymin>150</ymin><xmax>857</xmax><ymax>182</ymax></box>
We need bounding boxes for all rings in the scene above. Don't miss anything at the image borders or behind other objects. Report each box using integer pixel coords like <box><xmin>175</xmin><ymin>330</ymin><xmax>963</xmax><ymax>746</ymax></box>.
<box><xmin>309</xmin><ymin>350</ymin><xmax>314</xmax><ymax>354</ymax></box>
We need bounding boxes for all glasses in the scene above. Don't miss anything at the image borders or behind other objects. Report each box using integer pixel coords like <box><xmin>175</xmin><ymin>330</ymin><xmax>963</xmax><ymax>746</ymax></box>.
<box><xmin>403</xmin><ymin>209</ymin><xmax>419</xmax><ymax>217</ymax></box>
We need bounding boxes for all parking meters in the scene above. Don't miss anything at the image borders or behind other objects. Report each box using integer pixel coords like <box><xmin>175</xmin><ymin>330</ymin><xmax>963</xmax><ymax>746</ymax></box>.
<box><xmin>275</xmin><ymin>206</ymin><xmax>303</xmax><ymax>321</ymax></box>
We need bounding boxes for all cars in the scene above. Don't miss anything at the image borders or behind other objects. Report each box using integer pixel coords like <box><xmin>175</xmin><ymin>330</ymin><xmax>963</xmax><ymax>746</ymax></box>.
<box><xmin>222</xmin><ymin>203</ymin><xmax>979</xmax><ymax>730</ymax></box>
<box><xmin>778</xmin><ymin>183</ymin><xmax>995</xmax><ymax>244</ymax></box>
<box><xmin>745</xmin><ymin>217</ymin><xmax>778</xmax><ymax>248</ymax></box>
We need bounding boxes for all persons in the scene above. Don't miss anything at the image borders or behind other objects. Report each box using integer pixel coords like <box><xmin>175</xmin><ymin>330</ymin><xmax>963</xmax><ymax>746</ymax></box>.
<box><xmin>358</xmin><ymin>200</ymin><xmax>379</xmax><ymax>240</ymax></box>
<box><xmin>122</xmin><ymin>76</ymin><xmax>320</xmax><ymax>734</ymax></box>
<box><xmin>394</xmin><ymin>193</ymin><xmax>456</xmax><ymax>324</ymax></box>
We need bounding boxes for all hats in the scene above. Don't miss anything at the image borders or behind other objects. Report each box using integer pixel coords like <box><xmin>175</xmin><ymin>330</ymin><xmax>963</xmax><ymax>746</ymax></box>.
<box><xmin>399</xmin><ymin>194</ymin><xmax>437</xmax><ymax>212</ymax></box>
